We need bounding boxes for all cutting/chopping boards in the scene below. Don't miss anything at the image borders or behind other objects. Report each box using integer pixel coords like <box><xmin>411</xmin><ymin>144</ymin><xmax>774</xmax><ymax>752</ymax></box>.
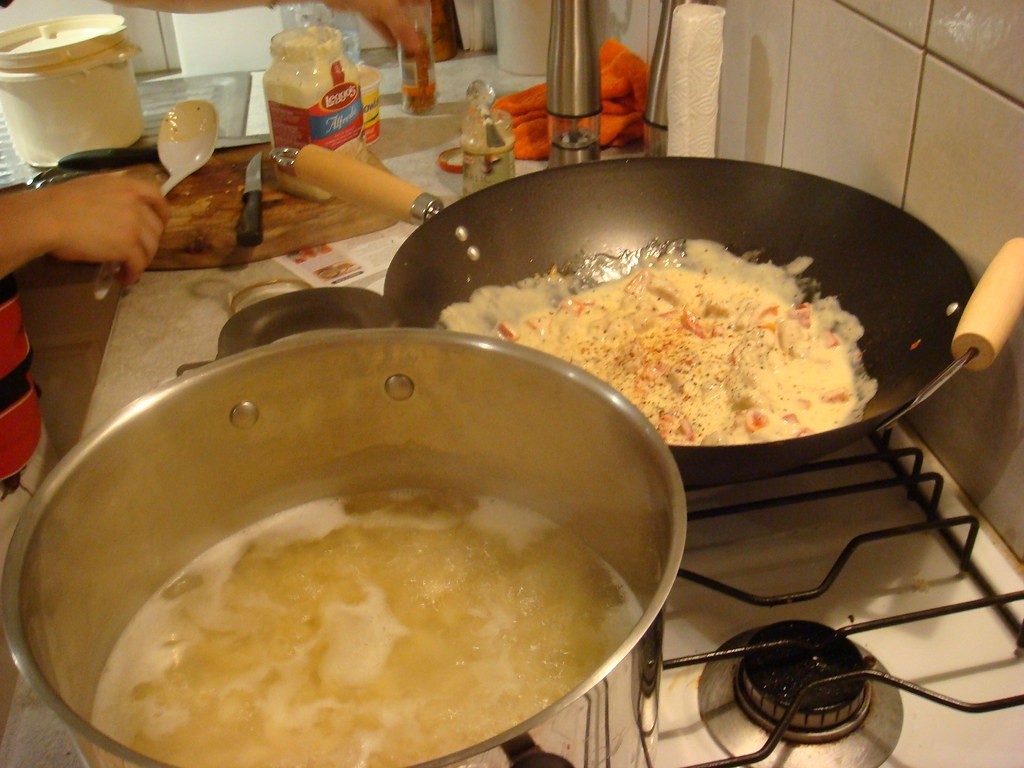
<box><xmin>114</xmin><ymin>142</ymin><xmax>402</xmax><ymax>269</ymax></box>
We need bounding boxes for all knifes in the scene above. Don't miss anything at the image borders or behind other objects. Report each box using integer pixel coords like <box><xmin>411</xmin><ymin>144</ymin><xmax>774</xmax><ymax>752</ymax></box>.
<box><xmin>236</xmin><ymin>152</ymin><xmax>263</xmax><ymax>249</ymax></box>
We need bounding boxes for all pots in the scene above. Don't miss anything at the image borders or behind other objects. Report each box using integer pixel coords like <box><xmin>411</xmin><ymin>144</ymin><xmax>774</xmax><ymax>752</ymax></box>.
<box><xmin>384</xmin><ymin>156</ymin><xmax>1024</xmax><ymax>486</ymax></box>
<box><xmin>0</xmin><ymin>14</ymin><xmax>144</xmax><ymax>168</ymax></box>
<box><xmin>176</xmin><ymin>278</ymin><xmax>384</xmax><ymax>381</ymax></box>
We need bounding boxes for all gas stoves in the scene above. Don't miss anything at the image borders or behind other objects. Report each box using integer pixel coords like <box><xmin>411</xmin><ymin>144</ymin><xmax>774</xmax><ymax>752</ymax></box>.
<box><xmin>584</xmin><ymin>404</ymin><xmax>1024</xmax><ymax>768</ymax></box>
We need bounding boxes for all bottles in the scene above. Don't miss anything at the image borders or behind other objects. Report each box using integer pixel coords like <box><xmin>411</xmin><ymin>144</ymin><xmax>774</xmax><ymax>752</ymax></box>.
<box><xmin>543</xmin><ymin>0</ymin><xmax>601</xmax><ymax>166</ymax></box>
<box><xmin>643</xmin><ymin>0</ymin><xmax>706</xmax><ymax>154</ymax></box>
<box><xmin>396</xmin><ymin>0</ymin><xmax>435</xmax><ymax>113</ymax></box>
<box><xmin>263</xmin><ymin>26</ymin><xmax>362</xmax><ymax>204</ymax></box>
<box><xmin>461</xmin><ymin>109</ymin><xmax>515</xmax><ymax>196</ymax></box>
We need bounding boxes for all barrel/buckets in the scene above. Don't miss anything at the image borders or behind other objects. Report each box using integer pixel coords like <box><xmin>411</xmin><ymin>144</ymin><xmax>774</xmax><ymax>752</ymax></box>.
<box><xmin>0</xmin><ymin>326</ymin><xmax>688</xmax><ymax>768</ymax></box>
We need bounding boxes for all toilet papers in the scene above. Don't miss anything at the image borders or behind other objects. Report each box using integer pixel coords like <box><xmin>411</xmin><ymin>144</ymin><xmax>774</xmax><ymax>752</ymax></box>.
<box><xmin>666</xmin><ymin>4</ymin><xmax>726</xmax><ymax>157</ymax></box>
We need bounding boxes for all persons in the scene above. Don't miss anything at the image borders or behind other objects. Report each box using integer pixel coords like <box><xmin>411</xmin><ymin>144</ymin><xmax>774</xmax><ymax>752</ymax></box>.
<box><xmin>0</xmin><ymin>0</ymin><xmax>422</xmax><ymax>591</ymax></box>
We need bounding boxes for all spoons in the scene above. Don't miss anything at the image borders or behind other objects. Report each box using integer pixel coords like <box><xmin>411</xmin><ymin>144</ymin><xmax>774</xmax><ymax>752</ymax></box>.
<box><xmin>93</xmin><ymin>100</ymin><xmax>219</xmax><ymax>301</ymax></box>
<box><xmin>465</xmin><ymin>79</ymin><xmax>506</xmax><ymax>148</ymax></box>
